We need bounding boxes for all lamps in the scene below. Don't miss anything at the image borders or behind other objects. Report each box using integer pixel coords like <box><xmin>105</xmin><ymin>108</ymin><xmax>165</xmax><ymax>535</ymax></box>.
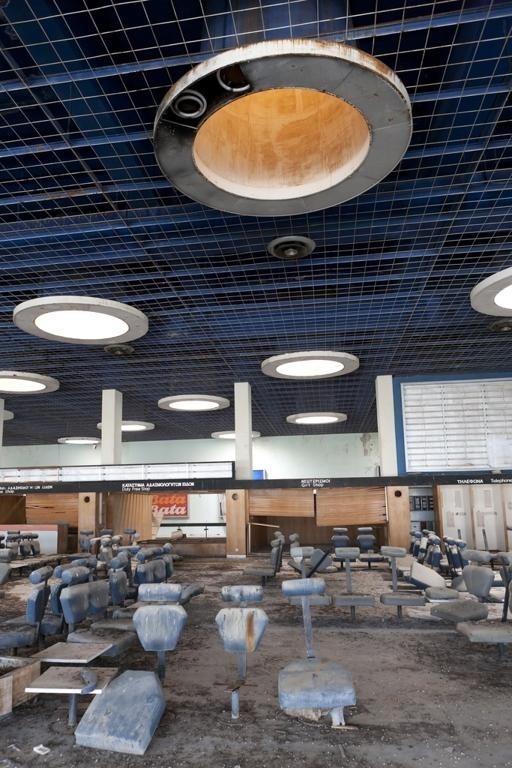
<box><xmin>0</xmin><ymin>370</ymin><xmax>59</xmax><ymax>395</ymax></box>
<box><xmin>261</xmin><ymin>350</ymin><xmax>360</xmax><ymax>380</ymax></box>
<box><xmin>469</xmin><ymin>266</ymin><xmax>512</xmax><ymax>317</ymax></box>
<box><xmin>286</xmin><ymin>412</ymin><xmax>347</xmax><ymax>425</ymax></box>
<box><xmin>152</xmin><ymin>40</ymin><xmax>414</xmax><ymax>217</ymax></box>
<box><xmin>57</xmin><ymin>436</ymin><xmax>100</xmax><ymax>444</ymax></box>
<box><xmin>158</xmin><ymin>394</ymin><xmax>230</xmax><ymax>412</ymax></box>
<box><xmin>97</xmin><ymin>421</ymin><xmax>155</xmax><ymax>432</ymax></box>
<box><xmin>13</xmin><ymin>296</ymin><xmax>149</xmax><ymax>346</ymax></box>
<box><xmin>210</xmin><ymin>431</ymin><xmax>261</xmax><ymax>440</ymax></box>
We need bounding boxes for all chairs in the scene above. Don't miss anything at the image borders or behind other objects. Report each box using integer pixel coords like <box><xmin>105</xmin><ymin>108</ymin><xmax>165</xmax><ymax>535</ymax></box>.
<box><xmin>72</xmin><ymin>581</ymin><xmax>189</xmax><ymax>754</ymax></box>
<box><xmin>232</xmin><ymin>526</ymin><xmax>511</xmax><ymax>647</ymax></box>
<box><xmin>274</xmin><ymin>576</ymin><xmax>359</xmax><ymax>730</ymax></box>
<box><xmin>1</xmin><ymin>526</ymin><xmax>183</xmax><ymax>697</ymax></box>
<box><xmin>215</xmin><ymin>581</ymin><xmax>269</xmax><ymax>725</ymax></box>
